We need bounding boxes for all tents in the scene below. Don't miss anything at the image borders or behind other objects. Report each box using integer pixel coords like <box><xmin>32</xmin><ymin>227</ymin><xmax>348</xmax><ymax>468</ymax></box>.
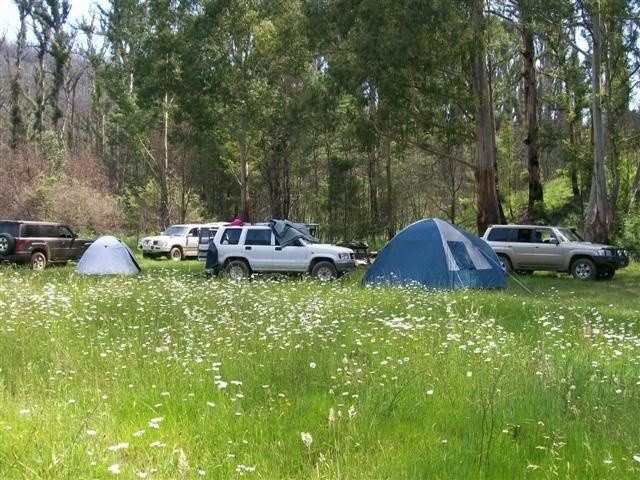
<box><xmin>363</xmin><ymin>218</ymin><xmax>508</xmax><ymax>291</ymax></box>
<box><xmin>76</xmin><ymin>235</ymin><xmax>141</xmax><ymax>276</ymax></box>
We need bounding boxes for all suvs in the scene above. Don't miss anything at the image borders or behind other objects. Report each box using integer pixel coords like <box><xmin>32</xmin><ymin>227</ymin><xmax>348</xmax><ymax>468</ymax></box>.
<box><xmin>0</xmin><ymin>218</ymin><xmax>96</xmax><ymax>272</ymax></box>
<box><xmin>205</xmin><ymin>224</ymin><xmax>357</xmax><ymax>288</ymax></box>
<box><xmin>478</xmin><ymin>222</ymin><xmax>631</xmax><ymax>283</ymax></box>
<box><xmin>136</xmin><ymin>222</ymin><xmax>212</xmax><ymax>262</ymax></box>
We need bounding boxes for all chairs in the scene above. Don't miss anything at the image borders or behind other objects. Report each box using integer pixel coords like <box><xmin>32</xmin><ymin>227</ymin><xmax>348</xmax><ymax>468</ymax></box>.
<box><xmin>519</xmin><ymin>230</ymin><xmax>542</xmax><ymax>242</ymax></box>
